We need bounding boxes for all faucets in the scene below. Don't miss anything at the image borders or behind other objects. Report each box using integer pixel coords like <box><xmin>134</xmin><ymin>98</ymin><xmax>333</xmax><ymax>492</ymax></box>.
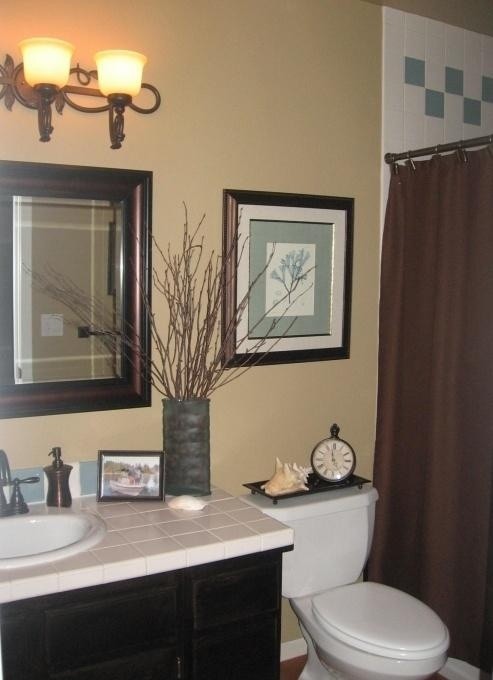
<box><xmin>0</xmin><ymin>450</ymin><xmax>12</xmax><ymax>486</ymax></box>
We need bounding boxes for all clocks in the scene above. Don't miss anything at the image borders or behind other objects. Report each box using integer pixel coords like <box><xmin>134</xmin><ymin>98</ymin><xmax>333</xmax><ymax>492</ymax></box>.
<box><xmin>309</xmin><ymin>424</ymin><xmax>357</xmax><ymax>488</ymax></box>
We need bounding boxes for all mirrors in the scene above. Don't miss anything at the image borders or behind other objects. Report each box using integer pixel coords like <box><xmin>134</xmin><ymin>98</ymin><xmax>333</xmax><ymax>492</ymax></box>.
<box><xmin>0</xmin><ymin>159</ymin><xmax>153</xmax><ymax>418</ymax></box>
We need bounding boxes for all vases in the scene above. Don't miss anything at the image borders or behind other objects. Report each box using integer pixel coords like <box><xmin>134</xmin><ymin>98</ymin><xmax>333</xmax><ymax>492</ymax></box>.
<box><xmin>162</xmin><ymin>399</ymin><xmax>210</xmax><ymax>496</ymax></box>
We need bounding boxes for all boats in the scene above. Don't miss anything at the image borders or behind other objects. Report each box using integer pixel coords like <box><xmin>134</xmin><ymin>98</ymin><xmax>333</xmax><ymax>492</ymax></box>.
<box><xmin>110</xmin><ymin>479</ymin><xmax>144</xmax><ymax>496</ymax></box>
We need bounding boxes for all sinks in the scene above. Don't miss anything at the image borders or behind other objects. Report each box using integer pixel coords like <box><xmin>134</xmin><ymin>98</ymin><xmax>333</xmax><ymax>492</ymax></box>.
<box><xmin>0</xmin><ymin>508</ymin><xmax>107</xmax><ymax>569</ymax></box>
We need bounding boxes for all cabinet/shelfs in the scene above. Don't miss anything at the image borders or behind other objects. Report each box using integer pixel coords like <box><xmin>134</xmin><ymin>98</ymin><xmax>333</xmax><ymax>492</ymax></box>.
<box><xmin>191</xmin><ymin>549</ymin><xmax>280</xmax><ymax>679</ymax></box>
<box><xmin>44</xmin><ymin>570</ymin><xmax>186</xmax><ymax>680</ymax></box>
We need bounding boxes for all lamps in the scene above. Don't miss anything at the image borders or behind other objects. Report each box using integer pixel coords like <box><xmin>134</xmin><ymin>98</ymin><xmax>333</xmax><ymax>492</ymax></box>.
<box><xmin>0</xmin><ymin>37</ymin><xmax>161</xmax><ymax>149</ymax></box>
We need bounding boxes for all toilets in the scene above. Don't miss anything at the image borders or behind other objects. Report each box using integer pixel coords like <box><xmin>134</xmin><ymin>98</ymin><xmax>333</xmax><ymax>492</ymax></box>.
<box><xmin>238</xmin><ymin>483</ymin><xmax>452</xmax><ymax>679</ymax></box>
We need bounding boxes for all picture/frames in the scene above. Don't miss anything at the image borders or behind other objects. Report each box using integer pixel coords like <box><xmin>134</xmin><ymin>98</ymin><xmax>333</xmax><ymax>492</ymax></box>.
<box><xmin>97</xmin><ymin>450</ymin><xmax>164</xmax><ymax>503</ymax></box>
<box><xmin>221</xmin><ymin>190</ymin><xmax>355</xmax><ymax>368</ymax></box>
